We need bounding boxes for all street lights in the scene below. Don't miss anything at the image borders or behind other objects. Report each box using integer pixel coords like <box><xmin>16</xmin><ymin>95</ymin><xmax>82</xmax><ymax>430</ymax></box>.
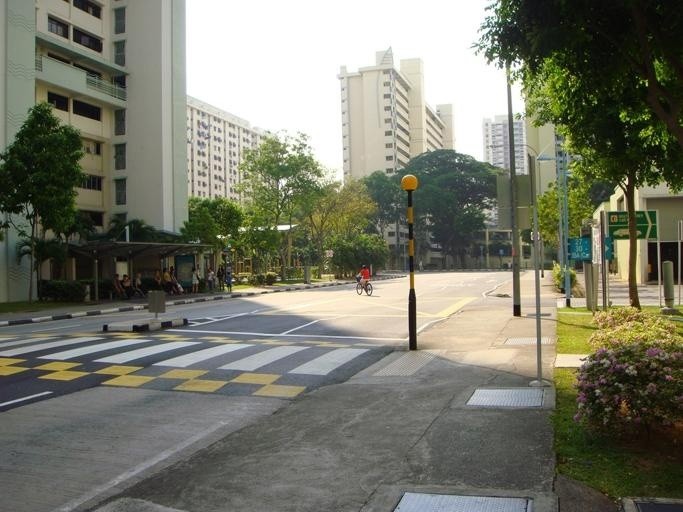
<box><xmin>486</xmin><ymin>143</ymin><xmax>542</xmax><ymax>194</ymax></box>
<box><xmin>400</xmin><ymin>174</ymin><xmax>418</xmax><ymax>350</ymax></box>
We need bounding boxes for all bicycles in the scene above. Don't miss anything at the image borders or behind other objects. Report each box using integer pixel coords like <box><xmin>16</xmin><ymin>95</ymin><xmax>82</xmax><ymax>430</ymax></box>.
<box><xmin>356</xmin><ymin>275</ymin><xmax>374</xmax><ymax>296</ymax></box>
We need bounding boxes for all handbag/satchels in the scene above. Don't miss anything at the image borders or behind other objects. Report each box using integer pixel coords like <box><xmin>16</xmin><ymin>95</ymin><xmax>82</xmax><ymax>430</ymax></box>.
<box><xmin>196</xmin><ymin>274</ymin><xmax>200</xmax><ymax>280</ymax></box>
<box><xmin>212</xmin><ymin>275</ymin><xmax>216</xmax><ymax>281</ymax></box>
<box><xmin>178</xmin><ymin>284</ymin><xmax>184</xmax><ymax>292</ymax></box>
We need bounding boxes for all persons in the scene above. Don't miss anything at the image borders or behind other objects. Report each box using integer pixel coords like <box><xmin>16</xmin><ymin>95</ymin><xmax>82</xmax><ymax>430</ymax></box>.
<box><xmin>111</xmin><ymin>265</ymin><xmax>232</xmax><ymax>300</ymax></box>
<box><xmin>356</xmin><ymin>264</ymin><xmax>369</xmax><ymax>291</ymax></box>
